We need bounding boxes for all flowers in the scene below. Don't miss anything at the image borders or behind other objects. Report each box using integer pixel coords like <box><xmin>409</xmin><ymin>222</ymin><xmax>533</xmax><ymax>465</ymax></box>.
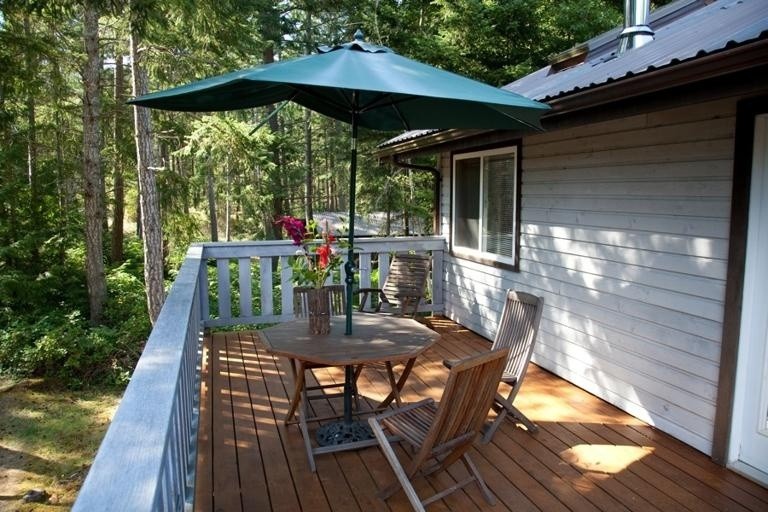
<box><xmin>271</xmin><ymin>209</ymin><xmax>367</xmax><ymax>292</ymax></box>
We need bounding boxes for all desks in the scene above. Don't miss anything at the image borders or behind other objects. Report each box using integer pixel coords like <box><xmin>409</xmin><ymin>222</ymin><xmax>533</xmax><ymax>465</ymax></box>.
<box><xmin>254</xmin><ymin>311</ymin><xmax>442</xmax><ymax>473</ymax></box>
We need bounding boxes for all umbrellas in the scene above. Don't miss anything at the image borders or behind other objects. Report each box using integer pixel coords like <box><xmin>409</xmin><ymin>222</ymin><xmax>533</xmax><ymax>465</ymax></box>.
<box><xmin>123</xmin><ymin>28</ymin><xmax>554</xmax><ymax>426</ymax></box>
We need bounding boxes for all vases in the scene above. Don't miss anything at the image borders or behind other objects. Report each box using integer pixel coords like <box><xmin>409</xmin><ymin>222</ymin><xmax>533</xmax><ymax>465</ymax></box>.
<box><xmin>305</xmin><ymin>285</ymin><xmax>335</xmax><ymax>337</ymax></box>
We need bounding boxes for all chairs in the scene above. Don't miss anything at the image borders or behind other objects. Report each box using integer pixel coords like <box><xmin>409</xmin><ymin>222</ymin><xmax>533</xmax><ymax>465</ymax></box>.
<box><xmin>440</xmin><ymin>284</ymin><xmax>550</xmax><ymax>448</ymax></box>
<box><xmin>367</xmin><ymin>342</ymin><xmax>515</xmax><ymax>512</ymax></box>
<box><xmin>281</xmin><ymin>282</ymin><xmax>363</xmax><ymax>429</ymax></box>
<box><xmin>347</xmin><ymin>252</ymin><xmax>435</xmax><ymax>413</ymax></box>
